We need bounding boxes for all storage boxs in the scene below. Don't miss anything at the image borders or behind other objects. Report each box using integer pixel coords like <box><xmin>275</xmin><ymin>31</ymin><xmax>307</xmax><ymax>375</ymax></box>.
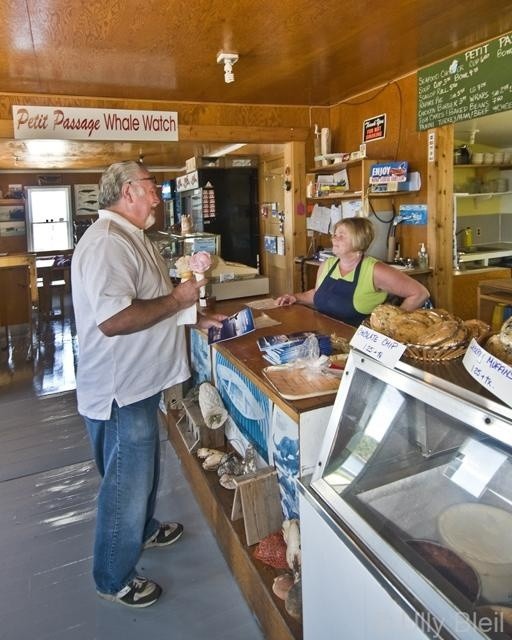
<box><xmin>185</xmin><ymin>154</ymin><xmax>257</xmax><ymax>173</ymax></box>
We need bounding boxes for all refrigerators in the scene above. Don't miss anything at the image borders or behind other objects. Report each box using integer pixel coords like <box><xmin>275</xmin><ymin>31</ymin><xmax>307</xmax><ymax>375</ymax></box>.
<box><xmin>161</xmin><ymin>167</ymin><xmax>261</xmax><ymax>269</ymax></box>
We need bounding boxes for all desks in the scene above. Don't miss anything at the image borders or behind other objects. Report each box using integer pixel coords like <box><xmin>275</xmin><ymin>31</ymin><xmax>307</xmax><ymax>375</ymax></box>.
<box><xmin>36</xmin><ymin>260</ymin><xmax>56</xmax><ymax>319</ymax></box>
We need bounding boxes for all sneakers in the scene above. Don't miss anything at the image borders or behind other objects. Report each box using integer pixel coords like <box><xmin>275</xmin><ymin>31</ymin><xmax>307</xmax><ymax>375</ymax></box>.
<box><xmin>94</xmin><ymin>573</ymin><xmax>163</xmax><ymax>610</ymax></box>
<box><xmin>141</xmin><ymin>520</ymin><xmax>184</xmax><ymax>550</ymax></box>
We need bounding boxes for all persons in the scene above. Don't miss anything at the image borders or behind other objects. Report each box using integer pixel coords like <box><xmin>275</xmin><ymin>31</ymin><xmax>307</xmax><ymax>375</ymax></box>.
<box><xmin>272</xmin><ymin>215</ymin><xmax>431</xmax><ymax>332</ymax></box>
<box><xmin>69</xmin><ymin>157</ymin><xmax>212</xmax><ymax>609</ymax></box>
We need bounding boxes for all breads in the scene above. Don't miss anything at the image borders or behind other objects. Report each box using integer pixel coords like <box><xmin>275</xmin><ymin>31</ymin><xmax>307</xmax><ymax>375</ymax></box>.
<box><xmin>370</xmin><ymin>303</ymin><xmax>512</xmax><ymax>366</ymax></box>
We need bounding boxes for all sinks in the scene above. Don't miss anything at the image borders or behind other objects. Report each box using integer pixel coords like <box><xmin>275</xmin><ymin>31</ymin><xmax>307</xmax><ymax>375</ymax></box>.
<box><xmin>385</xmin><ymin>261</ymin><xmax>415</xmax><ymax>270</ymax></box>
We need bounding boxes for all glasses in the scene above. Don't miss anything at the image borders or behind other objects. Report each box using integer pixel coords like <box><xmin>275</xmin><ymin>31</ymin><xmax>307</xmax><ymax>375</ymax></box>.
<box><xmin>125</xmin><ymin>173</ymin><xmax>160</xmax><ymax>186</ymax></box>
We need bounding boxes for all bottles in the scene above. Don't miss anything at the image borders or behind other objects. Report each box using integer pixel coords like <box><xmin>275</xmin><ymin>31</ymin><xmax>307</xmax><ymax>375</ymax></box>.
<box><xmin>465</xmin><ymin>226</ymin><xmax>472</xmax><ymax>246</ymax></box>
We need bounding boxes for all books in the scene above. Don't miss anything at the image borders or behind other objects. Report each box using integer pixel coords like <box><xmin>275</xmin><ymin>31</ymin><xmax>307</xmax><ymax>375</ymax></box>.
<box><xmin>256</xmin><ymin>328</ymin><xmax>332</xmax><ymax>365</ymax></box>
<box><xmin>208</xmin><ymin>307</ymin><xmax>256</xmax><ymax>345</ymax></box>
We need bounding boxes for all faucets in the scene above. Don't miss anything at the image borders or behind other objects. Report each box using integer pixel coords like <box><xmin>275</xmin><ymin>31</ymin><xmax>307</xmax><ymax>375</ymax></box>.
<box><xmin>393</xmin><ymin>257</ymin><xmax>415</xmax><ymax>267</ymax></box>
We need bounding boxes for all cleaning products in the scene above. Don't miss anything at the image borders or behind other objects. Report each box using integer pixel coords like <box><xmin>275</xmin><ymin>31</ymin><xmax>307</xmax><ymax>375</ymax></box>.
<box><xmin>463</xmin><ymin>227</ymin><xmax>473</xmax><ymax>247</ymax></box>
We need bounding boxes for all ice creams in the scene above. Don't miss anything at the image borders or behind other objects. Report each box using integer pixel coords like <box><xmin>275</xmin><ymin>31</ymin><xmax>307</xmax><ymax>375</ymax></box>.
<box><xmin>174</xmin><ymin>255</ymin><xmax>192</xmax><ymax>280</ymax></box>
<box><xmin>190</xmin><ymin>251</ymin><xmax>213</xmax><ymax>273</ymax></box>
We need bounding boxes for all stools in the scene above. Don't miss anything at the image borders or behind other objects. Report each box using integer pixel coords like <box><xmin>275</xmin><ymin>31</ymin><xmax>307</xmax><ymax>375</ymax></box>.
<box><xmin>35</xmin><ymin>280</ymin><xmax>69</xmax><ymax>321</ymax></box>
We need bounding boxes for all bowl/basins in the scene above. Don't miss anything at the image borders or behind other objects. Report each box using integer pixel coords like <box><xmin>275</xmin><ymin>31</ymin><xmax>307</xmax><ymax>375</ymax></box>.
<box><xmin>472</xmin><ymin>151</ymin><xmax>511</xmax><ymax>164</ymax></box>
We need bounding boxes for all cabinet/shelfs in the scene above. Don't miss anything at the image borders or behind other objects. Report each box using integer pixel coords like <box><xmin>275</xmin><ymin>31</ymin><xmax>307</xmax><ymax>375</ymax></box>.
<box><xmin>307</xmin><ymin>157</ymin><xmax>416</xmax><ymax>201</ymax></box>
<box><xmin>454</xmin><ymin>162</ymin><xmax>512</xmax><ymax>200</ymax></box>
